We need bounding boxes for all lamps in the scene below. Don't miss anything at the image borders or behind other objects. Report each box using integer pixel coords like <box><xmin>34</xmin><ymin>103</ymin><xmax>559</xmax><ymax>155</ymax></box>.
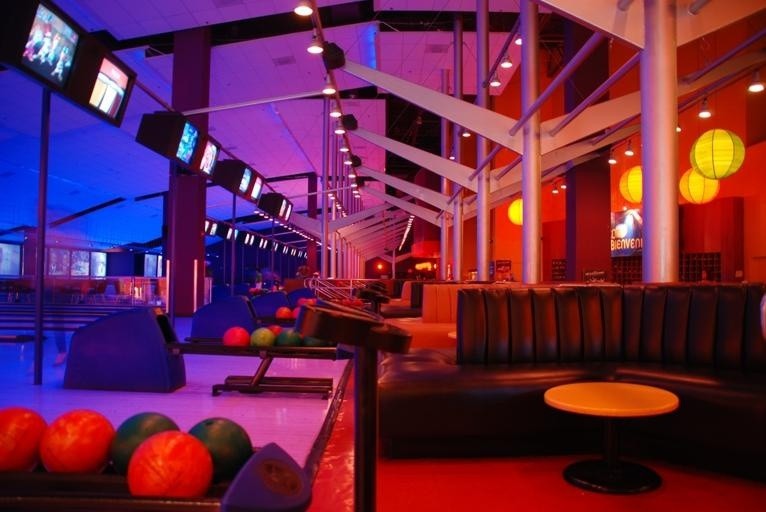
<box><xmin>457</xmin><ymin>15</ymin><xmax>522</xmax><ymax>137</ymax></box>
<box><xmin>550</xmin><ymin>61</ymin><xmax>766</xmax><ymax>193</ymax></box>
<box><xmin>294</xmin><ymin>0</ymin><xmax>361</xmax><ymax>218</ymax></box>
<box><xmin>678</xmin><ymin>90</ymin><xmax>746</xmax><ymax>204</ymax></box>
<box><xmin>619</xmin><ymin>165</ymin><xmax>643</xmax><ymax>203</ymax></box>
<box><xmin>507</xmin><ymin>198</ymin><xmax>523</xmax><ymax>225</ymax></box>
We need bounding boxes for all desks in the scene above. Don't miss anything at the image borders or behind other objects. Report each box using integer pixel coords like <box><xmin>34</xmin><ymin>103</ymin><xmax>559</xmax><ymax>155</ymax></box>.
<box><xmin>544</xmin><ymin>381</ymin><xmax>680</xmax><ymax>493</ymax></box>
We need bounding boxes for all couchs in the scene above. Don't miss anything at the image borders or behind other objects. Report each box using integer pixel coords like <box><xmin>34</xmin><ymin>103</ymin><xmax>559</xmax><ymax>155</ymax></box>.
<box><xmin>374</xmin><ymin>276</ymin><xmax>766</xmax><ymax>481</ymax></box>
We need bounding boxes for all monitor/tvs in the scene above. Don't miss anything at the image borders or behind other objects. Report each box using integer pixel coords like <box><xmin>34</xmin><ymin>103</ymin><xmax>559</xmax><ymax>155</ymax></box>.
<box><xmin>205</xmin><ymin>218</ymin><xmax>307</xmax><ymax>261</ymax></box>
<box><xmin>282</xmin><ymin>202</ymin><xmax>293</xmax><ymax>222</ymax></box>
<box><xmin>247</xmin><ymin>171</ymin><xmax>264</xmax><ymax>201</ymax></box>
<box><xmin>257</xmin><ymin>190</ymin><xmax>286</xmax><ymax>220</ymax></box>
<box><xmin>135</xmin><ymin>111</ymin><xmax>200</xmax><ymax>167</ymax></box>
<box><xmin>190</xmin><ymin>134</ymin><xmax>219</xmax><ymax>178</ymax></box>
<box><xmin>215</xmin><ymin>156</ymin><xmax>253</xmax><ymax>195</ymax></box>
<box><xmin>0</xmin><ymin>0</ymin><xmax>83</xmax><ymax>92</ymax></box>
<box><xmin>66</xmin><ymin>36</ymin><xmax>138</xmax><ymax>126</ymax></box>
